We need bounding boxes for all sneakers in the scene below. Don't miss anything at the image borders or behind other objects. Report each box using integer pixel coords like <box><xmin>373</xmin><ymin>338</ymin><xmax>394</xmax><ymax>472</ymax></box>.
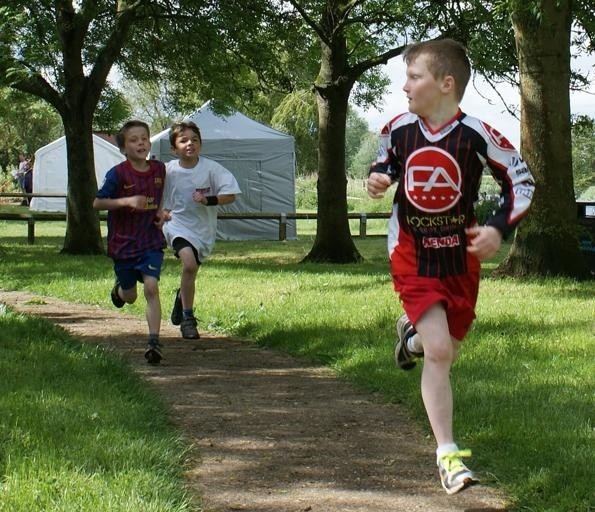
<box><xmin>111</xmin><ymin>270</ymin><xmax>127</xmax><ymax>308</ymax></box>
<box><xmin>394</xmin><ymin>313</ymin><xmax>418</xmax><ymax>370</ymax></box>
<box><xmin>437</xmin><ymin>444</ymin><xmax>480</xmax><ymax>495</ymax></box>
<box><xmin>144</xmin><ymin>337</ymin><xmax>163</xmax><ymax>365</ymax></box>
<box><xmin>170</xmin><ymin>288</ymin><xmax>184</xmax><ymax>325</ymax></box>
<box><xmin>180</xmin><ymin>317</ymin><xmax>200</xmax><ymax>340</ymax></box>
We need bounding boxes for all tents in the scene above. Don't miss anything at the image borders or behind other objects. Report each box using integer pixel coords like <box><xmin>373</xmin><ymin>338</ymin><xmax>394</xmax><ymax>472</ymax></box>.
<box><xmin>147</xmin><ymin>96</ymin><xmax>298</xmax><ymax>242</ymax></box>
<box><xmin>28</xmin><ymin>134</ymin><xmax>127</xmax><ymax>213</ymax></box>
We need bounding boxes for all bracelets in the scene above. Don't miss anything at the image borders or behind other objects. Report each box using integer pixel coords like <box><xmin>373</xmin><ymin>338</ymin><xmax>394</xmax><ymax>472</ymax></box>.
<box><xmin>204</xmin><ymin>194</ymin><xmax>219</xmax><ymax>207</ymax></box>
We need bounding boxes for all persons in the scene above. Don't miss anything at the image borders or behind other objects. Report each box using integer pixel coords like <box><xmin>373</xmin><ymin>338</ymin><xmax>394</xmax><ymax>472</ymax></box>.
<box><xmin>161</xmin><ymin>119</ymin><xmax>244</xmax><ymax>341</ymax></box>
<box><xmin>365</xmin><ymin>35</ymin><xmax>538</xmax><ymax>497</ymax></box>
<box><xmin>16</xmin><ymin>151</ymin><xmax>30</xmax><ymax>206</ymax></box>
<box><xmin>91</xmin><ymin>118</ymin><xmax>169</xmax><ymax>365</ymax></box>
